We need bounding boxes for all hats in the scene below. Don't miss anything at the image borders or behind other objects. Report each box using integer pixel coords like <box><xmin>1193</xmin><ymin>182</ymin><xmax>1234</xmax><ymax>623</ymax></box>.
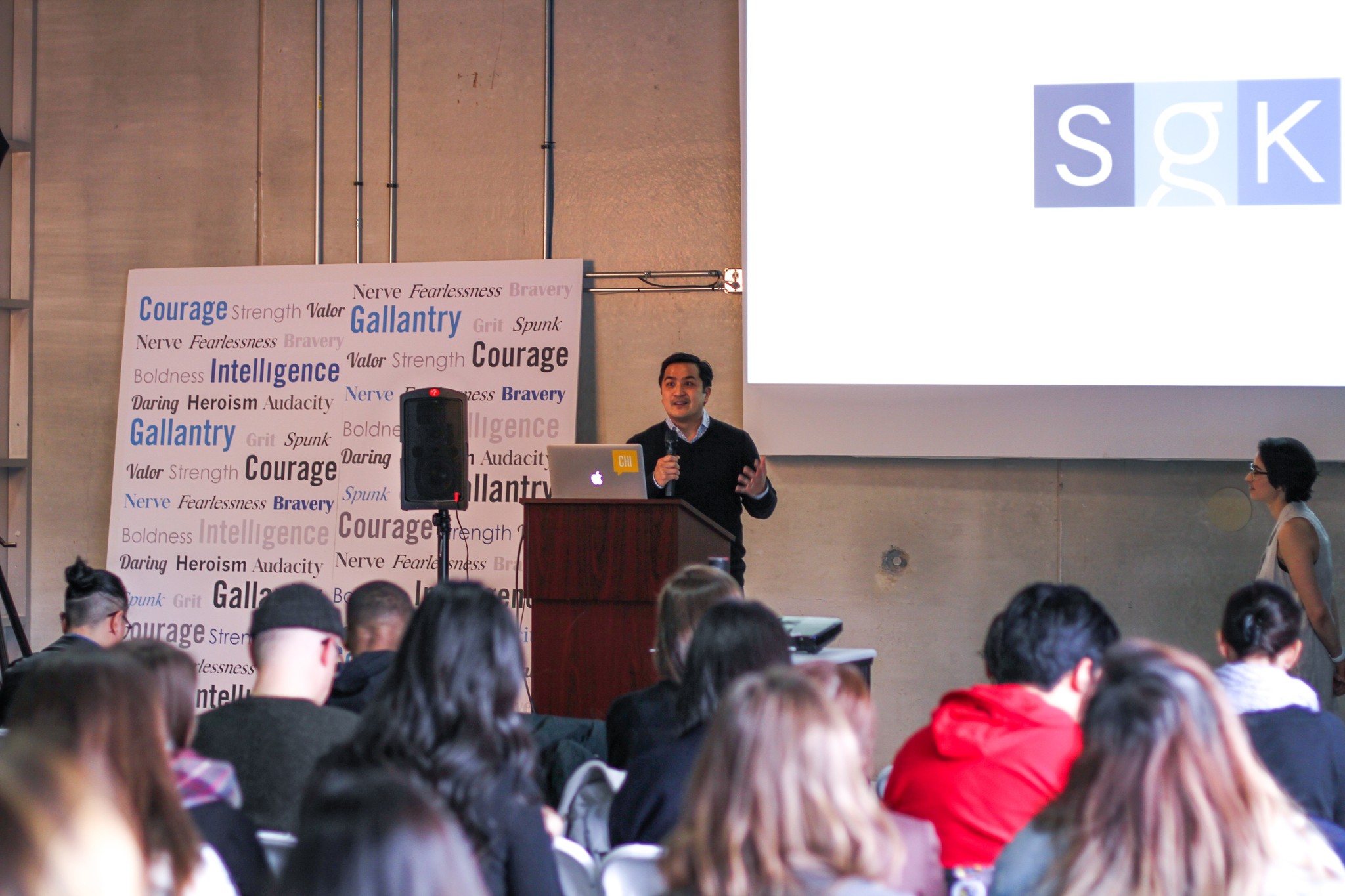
<box><xmin>251</xmin><ymin>583</ymin><xmax>345</xmax><ymax>639</ymax></box>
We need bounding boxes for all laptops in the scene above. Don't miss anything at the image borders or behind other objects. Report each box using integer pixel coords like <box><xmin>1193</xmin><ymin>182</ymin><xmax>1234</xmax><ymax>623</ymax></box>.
<box><xmin>546</xmin><ymin>444</ymin><xmax>648</xmax><ymax>498</ymax></box>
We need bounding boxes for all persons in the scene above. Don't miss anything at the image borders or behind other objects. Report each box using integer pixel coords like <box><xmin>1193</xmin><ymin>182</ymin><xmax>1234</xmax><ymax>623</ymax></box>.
<box><xmin>624</xmin><ymin>352</ymin><xmax>777</xmax><ymax>599</ymax></box>
<box><xmin>1245</xmin><ymin>437</ymin><xmax>1345</xmax><ymax>716</ymax></box>
<box><xmin>0</xmin><ymin>556</ymin><xmax>1345</xmax><ymax>896</ymax></box>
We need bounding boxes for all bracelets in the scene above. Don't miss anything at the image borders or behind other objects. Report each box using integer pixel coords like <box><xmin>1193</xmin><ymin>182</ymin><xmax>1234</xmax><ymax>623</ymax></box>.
<box><xmin>1328</xmin><ymin>647</ymin><xmax>1345</xmax><ymax>663</ymax></box>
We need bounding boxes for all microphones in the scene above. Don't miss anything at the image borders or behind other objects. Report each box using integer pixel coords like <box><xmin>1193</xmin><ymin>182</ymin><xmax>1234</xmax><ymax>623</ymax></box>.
<box><xmin>665</xmin><ymin>430</ymin><xmax>677</xmax><ymax>496</ymax></box>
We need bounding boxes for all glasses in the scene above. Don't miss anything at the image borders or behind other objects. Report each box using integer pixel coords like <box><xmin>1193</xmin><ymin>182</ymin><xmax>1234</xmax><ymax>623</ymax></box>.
<box><xmin>1251</xmin><ymin>463</ymin><xmax>1268</xmax><ymax>481</ymax></box>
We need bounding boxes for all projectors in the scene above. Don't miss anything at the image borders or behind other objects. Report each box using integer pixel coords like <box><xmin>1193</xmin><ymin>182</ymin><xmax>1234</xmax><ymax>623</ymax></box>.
<box><xmin>777</xmin><ymin>616</ymin><xmax>842</xmax><ymax>653</ymax></box>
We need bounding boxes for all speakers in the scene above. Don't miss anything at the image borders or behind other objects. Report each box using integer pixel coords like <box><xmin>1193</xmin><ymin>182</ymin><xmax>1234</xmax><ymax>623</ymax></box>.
<box><xmin>398</xmin><ymin>387</ymin><xmax>469</xmax><ymax>511</ymax></box>
